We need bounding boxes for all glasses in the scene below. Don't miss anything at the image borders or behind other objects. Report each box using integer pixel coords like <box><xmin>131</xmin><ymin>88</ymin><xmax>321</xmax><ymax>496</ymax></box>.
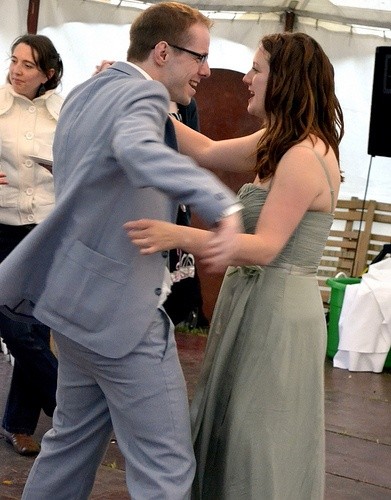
<box><xmin>151</xmin><ymin>42</ymin><xmax>209</xmax><ymax>65</ymax></box>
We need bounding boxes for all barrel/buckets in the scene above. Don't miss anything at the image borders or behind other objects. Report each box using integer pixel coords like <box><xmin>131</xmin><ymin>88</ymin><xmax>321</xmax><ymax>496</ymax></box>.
<box><xmin>325</xmin><ymin>276</ymin><xmax>391</xmax><ymax>372</ymax></box>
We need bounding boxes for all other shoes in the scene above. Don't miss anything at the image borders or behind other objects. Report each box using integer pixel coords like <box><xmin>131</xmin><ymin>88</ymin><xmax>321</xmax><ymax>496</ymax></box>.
<box><xmin>3</xmin><ymin>428</ymin><xmax>41</xmax><ymax>455</ymax></box>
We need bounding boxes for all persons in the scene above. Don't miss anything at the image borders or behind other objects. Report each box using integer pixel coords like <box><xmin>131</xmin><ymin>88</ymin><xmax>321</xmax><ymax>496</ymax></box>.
<box><xmin>1</xmin><ymin>34</ymin><xmax>88</xmax><ymax>455</ymax></box>
<box><xmin>94</xmin><ymin>31</ymin><xmax>345</xmax><ymax>500</ymax></box>
<box><xmin>0</xmin><ymin>2</ymin><xmax>245</xmax><ymax>500</ymax></box>
<box><xmin>148</xmin><ymin>75</ymin><xmax>211</xmax><ymax>339</ymax></box>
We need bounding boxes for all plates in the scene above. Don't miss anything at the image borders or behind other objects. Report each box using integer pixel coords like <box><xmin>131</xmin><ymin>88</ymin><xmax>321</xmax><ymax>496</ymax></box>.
<box><xmin>29</xmin><ymin>155</ymin><xmax>53</xmax><ymax>166</ymax></box>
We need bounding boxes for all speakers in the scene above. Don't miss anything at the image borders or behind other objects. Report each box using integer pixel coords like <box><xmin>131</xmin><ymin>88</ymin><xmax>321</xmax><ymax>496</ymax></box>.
<box><xmin>368</xmin><ymin>46</ymin><xmax>391</xmax><ymax>157</ymax></box>
